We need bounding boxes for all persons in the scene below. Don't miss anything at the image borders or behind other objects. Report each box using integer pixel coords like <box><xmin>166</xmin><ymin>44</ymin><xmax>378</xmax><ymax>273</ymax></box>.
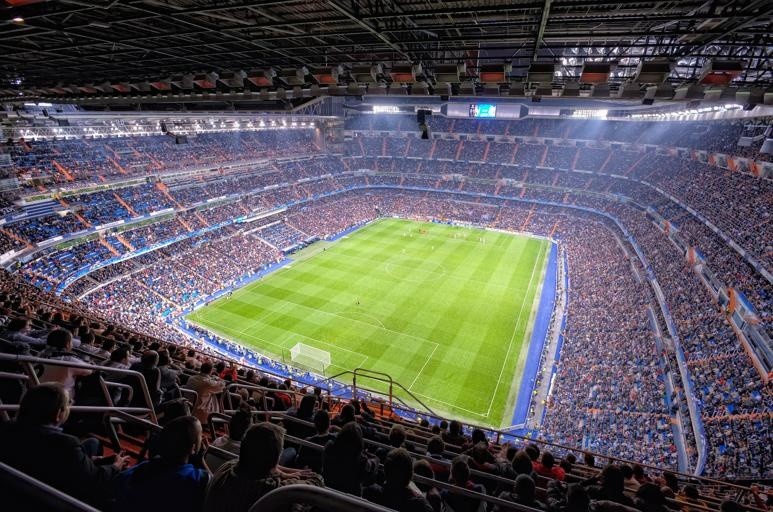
<box><xmin>0</xmin><ymin>108</ymin><xmax>772</xmax><ymax>512</ymax></box>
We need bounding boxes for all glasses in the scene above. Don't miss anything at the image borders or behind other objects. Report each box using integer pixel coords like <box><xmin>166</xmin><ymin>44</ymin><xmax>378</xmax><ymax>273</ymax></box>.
<box><xmin>60</xmin><ymin>397</ymin><xmax>75</xmax><ymax>410</ymax></box>
